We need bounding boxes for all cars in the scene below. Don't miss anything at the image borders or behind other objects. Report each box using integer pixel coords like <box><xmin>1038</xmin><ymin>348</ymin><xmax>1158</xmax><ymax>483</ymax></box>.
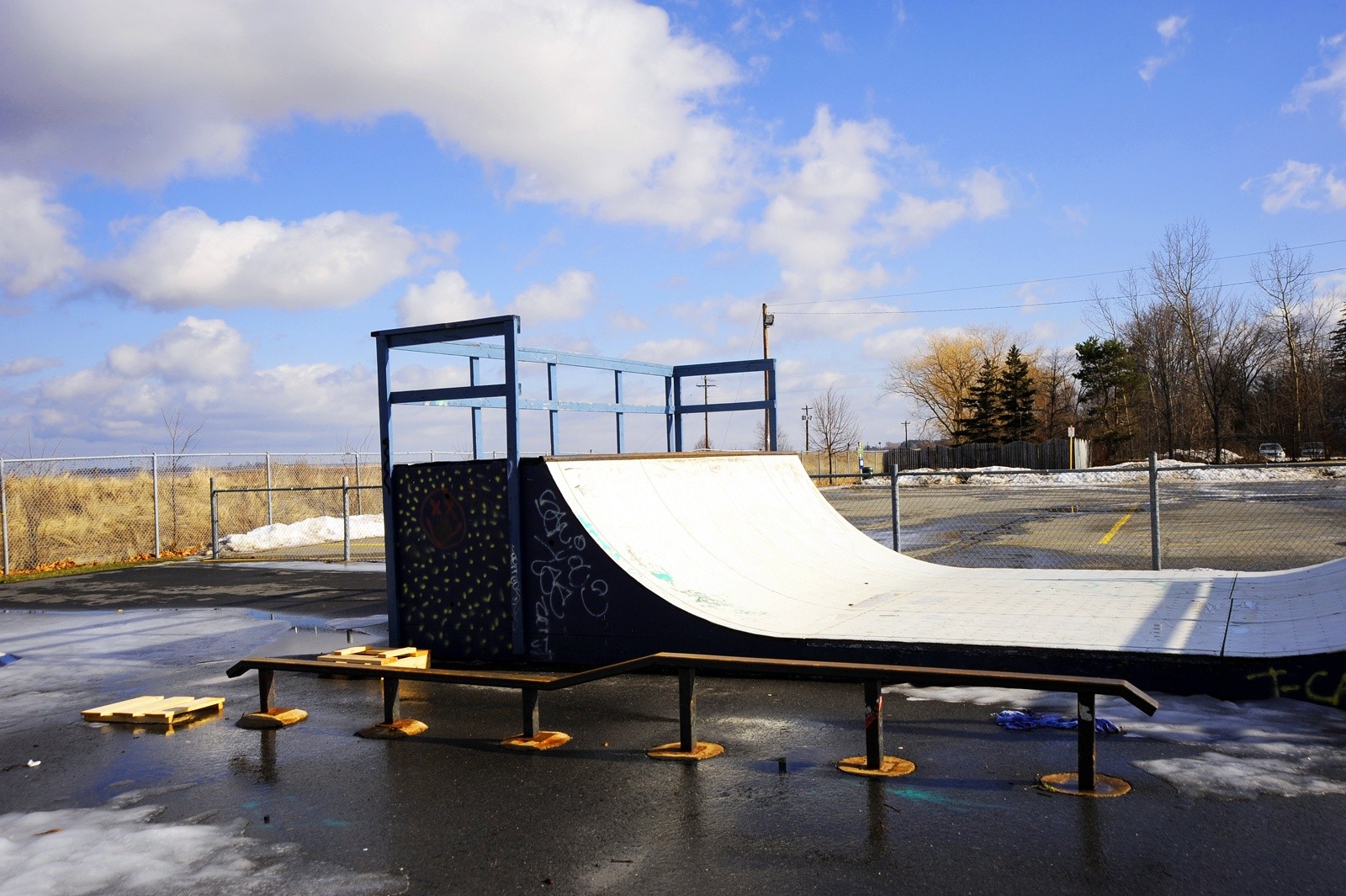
<box><xmin>1300</xmin><ymin>442</ymin><xmax>1331</xmax><ymax>457</ymax></box>
<box><xmin>1258</xmin><ymin>443</ymin><xmax>1286</xmax><ymax>461</ymax></box>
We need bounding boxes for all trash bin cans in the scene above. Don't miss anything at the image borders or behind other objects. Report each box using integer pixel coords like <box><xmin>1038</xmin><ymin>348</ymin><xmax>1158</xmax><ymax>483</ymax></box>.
<box><xmin>862</xmin><ymin>466</ymin><xmax>873</xmax><ymax>480</ymax></box>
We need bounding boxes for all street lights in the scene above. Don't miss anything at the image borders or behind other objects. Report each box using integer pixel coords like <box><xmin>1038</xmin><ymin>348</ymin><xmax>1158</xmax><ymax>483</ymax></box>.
<box><xmin>763</xmin><ymin>314</ymin><xmax>775</xmax><ymax>451</ymax></box>
